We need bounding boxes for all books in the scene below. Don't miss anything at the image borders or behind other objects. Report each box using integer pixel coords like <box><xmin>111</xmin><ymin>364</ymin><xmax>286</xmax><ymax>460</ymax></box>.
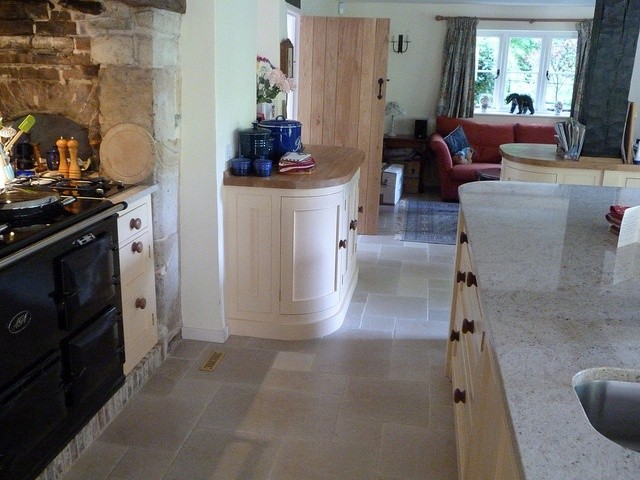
<box><xmin>621</xmin><ymin>101</ymin><xmax>636</xmax><ymax>164</ymax></box>
<box><xmin>281</xmin><ymin>151</ymin><xmax>312</xmax><ymax>162</ymax></box>
<box><xmin>553</xmin><ymin>111</ymin><xmax>591</xmax><ymax>160</ymax></box>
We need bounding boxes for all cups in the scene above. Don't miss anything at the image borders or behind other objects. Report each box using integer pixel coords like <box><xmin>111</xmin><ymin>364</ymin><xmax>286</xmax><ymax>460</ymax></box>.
<box><xmin>253</xmin><ymin>155</ymin><xmax>273</xmax><ymax>177</ymax></box>
<box><xmin>231</xmin><ymin>154</ymin><xmax>253</xmax><ymax>176</ymax></box>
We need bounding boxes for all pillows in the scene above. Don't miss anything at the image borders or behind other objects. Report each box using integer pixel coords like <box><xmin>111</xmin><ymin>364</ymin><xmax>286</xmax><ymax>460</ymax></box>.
<box><xmin>443</xmin><ymin>122</ymin><xmax>470</xmax><ymax>157</ymax></box>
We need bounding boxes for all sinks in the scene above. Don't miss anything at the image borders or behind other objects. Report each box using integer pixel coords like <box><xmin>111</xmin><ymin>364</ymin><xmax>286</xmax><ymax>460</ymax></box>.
<box><xmin>571</xmin><ymin>366</ymin><xmax>640</xmax><ymax>453</ymax></box>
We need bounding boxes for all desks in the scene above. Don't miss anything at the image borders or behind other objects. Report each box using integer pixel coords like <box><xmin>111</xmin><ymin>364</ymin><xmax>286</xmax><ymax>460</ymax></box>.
<box><xmin>383</xmin><ymin>132</ymin><xmax>428</xmax><ymax>194</ymax></box>
<box><xmin>499</xmin><ymin>143</ymin><xmax>640</xmax><ymax>188</ymax></box>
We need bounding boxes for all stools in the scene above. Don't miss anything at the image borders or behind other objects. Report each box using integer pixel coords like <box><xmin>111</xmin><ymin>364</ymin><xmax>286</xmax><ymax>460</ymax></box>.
<box><xmin>479</xmin><ymin>169</ymin><xmax>502</xmax><ymax>182</ymax></box>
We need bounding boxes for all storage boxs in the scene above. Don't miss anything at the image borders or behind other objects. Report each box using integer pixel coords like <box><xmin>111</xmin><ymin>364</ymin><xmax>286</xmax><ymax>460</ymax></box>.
<box><xmin>404</xmin><ymin>178</ymin><xmax>419</xmax><ymax>194</ymax></box>
<box><xmin>392</xmin><ymin>161</ymin><xmax>420</xmax><ymax>178</ymax></box>
<box><xmin>379</xmin><ymin>162</ymin><xmax>404</xmax><ymax>206</ymax></box>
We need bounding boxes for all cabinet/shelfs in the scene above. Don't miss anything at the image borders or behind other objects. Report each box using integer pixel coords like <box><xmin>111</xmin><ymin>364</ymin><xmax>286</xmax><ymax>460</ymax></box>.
<box><xmin>444</xmin><ymin>202</ymin><xmax>522</xmax><ymax>480</ymax></box>
<box><xmin>223</xmin><ymin>167</ymin><xmax>363</xmax><ymax>340</ymax></box>
<box><xmin>115</xmin><ymin>192</ymin><xmax>158</xmax><ymax>377</ymax></box>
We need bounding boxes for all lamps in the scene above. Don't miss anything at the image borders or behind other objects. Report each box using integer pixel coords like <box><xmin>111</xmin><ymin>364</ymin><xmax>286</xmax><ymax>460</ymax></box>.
<box><xmin>385</xmin><ymin>101</ymin><xmax>408</xmax><ymax>138</ymax></box>
<box><xmin>389</xmin><ymin>33</ymin><xmax>411</xmax><ymax>53</ymax></box>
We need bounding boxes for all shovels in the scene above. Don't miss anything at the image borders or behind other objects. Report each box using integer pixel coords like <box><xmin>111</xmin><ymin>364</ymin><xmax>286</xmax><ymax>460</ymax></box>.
<box><xmin>6</xmin><ymin>115</ymin><xmax>35</xmax><ymax>149</ymax></box>
<box><xmin>6</xmin><ymin>121</ymin><xmax>32</xmax><ymax>151</ymax></box>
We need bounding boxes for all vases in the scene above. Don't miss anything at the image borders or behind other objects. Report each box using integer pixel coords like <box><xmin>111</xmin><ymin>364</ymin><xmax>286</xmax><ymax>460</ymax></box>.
<box><xmin>257</xmin><ymin>102</ymin><xmax>272</xmax><ymax>121</ymax></box>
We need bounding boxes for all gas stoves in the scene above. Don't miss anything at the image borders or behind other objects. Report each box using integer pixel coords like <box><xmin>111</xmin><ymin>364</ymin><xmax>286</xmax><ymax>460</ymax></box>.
<box><xmin>4</xmin><ymin>175</ymin><xmax>136</xmax><ymax>199</ymax></box>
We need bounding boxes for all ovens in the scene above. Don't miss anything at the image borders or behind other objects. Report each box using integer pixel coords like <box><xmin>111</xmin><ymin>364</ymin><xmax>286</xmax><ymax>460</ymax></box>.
<box><xmin>0</xmin><ymin>201</ymin><xmax>129</xmax><ymax>480</ymax></box>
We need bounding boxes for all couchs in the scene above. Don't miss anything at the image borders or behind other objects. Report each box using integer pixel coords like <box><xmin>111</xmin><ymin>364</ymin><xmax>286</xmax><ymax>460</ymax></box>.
<box><xmin>430</xmin><ymin>114</ymin><xmax>561</xmax><ymax>201</ymax></box>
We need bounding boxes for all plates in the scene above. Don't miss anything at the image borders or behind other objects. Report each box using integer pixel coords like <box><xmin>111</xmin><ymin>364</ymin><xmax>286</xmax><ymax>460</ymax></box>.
<box><xmin>98</xmin><ymin>122</ymin><xmax>158</xmax><ymax>185</ymax></box>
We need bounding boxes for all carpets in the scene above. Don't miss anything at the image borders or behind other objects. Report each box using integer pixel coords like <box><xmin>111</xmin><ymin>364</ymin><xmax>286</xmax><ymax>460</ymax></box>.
<box><xmin>393</xmin><ymin>200</ymin><xmax>459</xmax><ymax>245</ymax></box>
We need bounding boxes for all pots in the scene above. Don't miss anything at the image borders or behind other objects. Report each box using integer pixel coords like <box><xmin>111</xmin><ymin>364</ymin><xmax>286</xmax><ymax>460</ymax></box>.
<box><xmin>258</xmin><ymin>115</ymin><xmax>303</xmax><ymax>157</ymax></box>
<box><xmin>239</xmin><ymin>121</ymin><xmax>276</xmax><ymax>160</ymax></box>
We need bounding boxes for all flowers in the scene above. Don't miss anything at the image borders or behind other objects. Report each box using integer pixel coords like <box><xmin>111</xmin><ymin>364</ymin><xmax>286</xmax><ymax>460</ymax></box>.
<box><xmin>256</xmin><ymin>56</ymin><xmax>297</xmax><ymax>104</ymax></box>
